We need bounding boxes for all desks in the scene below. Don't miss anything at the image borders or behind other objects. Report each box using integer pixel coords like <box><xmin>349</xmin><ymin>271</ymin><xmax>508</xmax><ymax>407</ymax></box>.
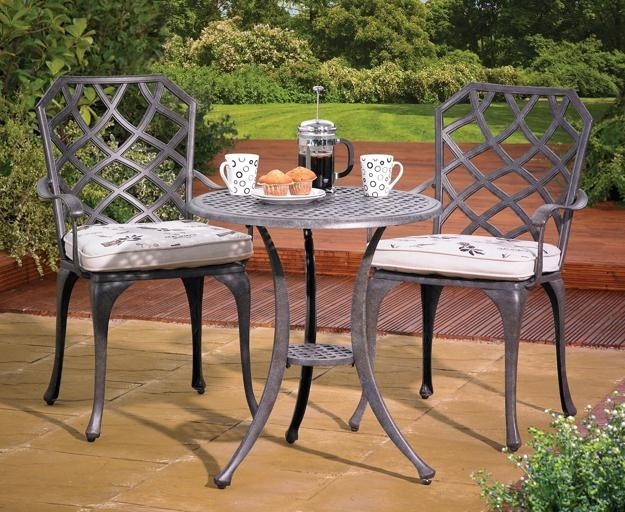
<box><xmin>190</xmin><ymin>186</ymin><xmax>439</xmax><ymax>489</ymax></box>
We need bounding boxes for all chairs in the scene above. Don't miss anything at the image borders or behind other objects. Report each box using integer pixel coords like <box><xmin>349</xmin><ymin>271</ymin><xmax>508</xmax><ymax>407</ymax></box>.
<box><xmin>348</xmin><ymin>80</ymin><xmax>596</xmax><ymax>451</ymax></box>
<box><xmin>34</xmin><ymin>74</ymin><xmax>262</xmax><ymax>443</ymax></box>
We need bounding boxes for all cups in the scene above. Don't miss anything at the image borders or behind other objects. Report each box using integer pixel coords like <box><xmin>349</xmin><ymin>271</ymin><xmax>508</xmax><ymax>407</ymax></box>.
<box><xmin>219</xmin><ymin>152</ymin><xmax>260</xmax><ymax>196</ymax></box>
<box><xmin>360</xmin><ymin>153</ymin><xmax>404</xmax><ymax>199</ymax></box>
<box><xmin>297</xmin><ymin>118</ymin><xmax>355</xmax><ymax>193</ymax></box>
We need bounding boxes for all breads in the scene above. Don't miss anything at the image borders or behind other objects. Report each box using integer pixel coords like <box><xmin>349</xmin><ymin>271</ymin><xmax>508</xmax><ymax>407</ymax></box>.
<box><xmin>286</xmin><ymin>166</ymin><xmax>317</xmax><ymax>196</ymax></box>
<box><xmin>258</xmin><ymin>169</ymin><xmax>293</xmax><ymax>196</ymax></box>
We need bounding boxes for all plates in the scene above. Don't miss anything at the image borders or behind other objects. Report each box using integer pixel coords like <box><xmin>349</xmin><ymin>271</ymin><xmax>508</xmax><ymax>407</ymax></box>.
<box><xmin>250</xmin><ymin>185</ymin><xmax>326</xmax><ymax>206</ymax></box>
